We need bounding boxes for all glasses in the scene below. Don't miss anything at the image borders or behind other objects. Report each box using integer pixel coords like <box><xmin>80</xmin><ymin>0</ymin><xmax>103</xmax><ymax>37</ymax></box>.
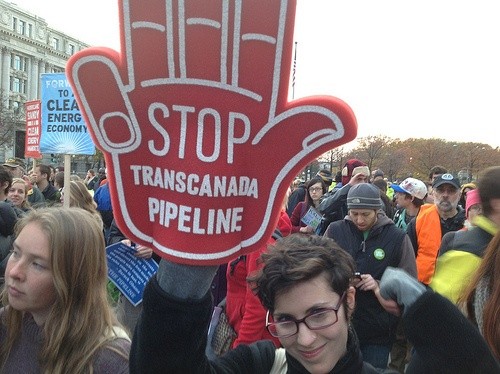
<box><xmin>308</xmin><ymin>186</ymin><xmax>323</xmax><ymax>192</ymax></box>
<box><xmin>265</xmin><ymin>290</ymin><xmax>347</xmax><ymax>339</ymax></box>
<box><xmin>99</xmin><ymin>172</ymin><xmax>105</xmax><ymax>174</ymax></box>
<box><xmin>469</xmin><ymin>205</ymin><xmax>484</xmax><ymax>212</ymax></box>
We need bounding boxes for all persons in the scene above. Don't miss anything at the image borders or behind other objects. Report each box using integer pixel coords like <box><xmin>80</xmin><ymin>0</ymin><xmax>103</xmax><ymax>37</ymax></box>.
<box><xmin>1</xmin><ymin>154</ymin><xmax>500</xmax><ymax>374</ymax></box>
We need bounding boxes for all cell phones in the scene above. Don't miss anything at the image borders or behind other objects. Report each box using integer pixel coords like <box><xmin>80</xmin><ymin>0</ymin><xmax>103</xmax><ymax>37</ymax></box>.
<box><xmin>352</xmin><ymin>272</ymin><xmax>362</xmax><ymax>281</ymax></box>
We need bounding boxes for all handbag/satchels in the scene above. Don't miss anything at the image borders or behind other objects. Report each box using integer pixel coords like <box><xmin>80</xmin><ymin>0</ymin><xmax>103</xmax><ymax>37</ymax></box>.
<box><xmin>211</xmin><ymin>312</ymin><xmax>238</xmax><ymax>358</ymax></box>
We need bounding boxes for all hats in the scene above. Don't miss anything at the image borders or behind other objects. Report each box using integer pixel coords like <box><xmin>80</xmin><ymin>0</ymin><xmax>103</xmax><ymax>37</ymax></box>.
<box><xmin>2</xmin><ymin>158</ymin><xmax>26</xmax><ymax>171</ymax></box>
<box><xmin>342</xmin><ymin>159</ymin><xmax>371</xmax><ymax>185</ymax></box>
<box><xmin>347</xmin><ymin>183</ymin><xmax>382</xmax><ymax>209</ymax></box>
<box><xmin>466</xmin><ymin>189</ymin><xmax>482</xmax><ymax>219</ymax></box>
<box><xmin>432</xmin><ymin>172</ymin><xmax>460</xmax><ymax>190</ymax></box>
<box><xmin>390</xmin><ymin>178</ymin><xmax>428</xmax><ymax>201</ymax></box>
